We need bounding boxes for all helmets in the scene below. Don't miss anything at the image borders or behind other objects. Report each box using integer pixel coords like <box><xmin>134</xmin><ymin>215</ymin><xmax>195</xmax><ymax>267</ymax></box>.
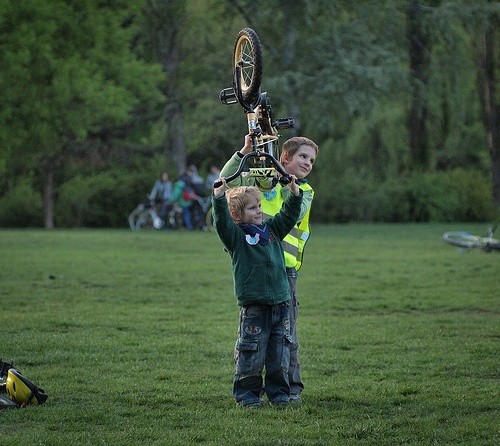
<box><xmin>6</xmin><ymin>369</ymin><xmax>48</xmax><ymax>404</ymax></box>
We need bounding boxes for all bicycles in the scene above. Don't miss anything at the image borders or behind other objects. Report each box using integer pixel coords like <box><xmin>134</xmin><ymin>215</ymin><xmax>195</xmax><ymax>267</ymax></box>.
<box><xmin>212</xmin><ymin>27</ymin><xmax>308</xmax><ymax>188</ymax></box>
<box><xmin>128</xmin><ymin>193</ymin><xmax>217</xmax><ymax>234</ymax></box>
<box><xmin>442</xmin><ymin>223</ymin><xmax>500</xmax><ymax>254</ymax></box>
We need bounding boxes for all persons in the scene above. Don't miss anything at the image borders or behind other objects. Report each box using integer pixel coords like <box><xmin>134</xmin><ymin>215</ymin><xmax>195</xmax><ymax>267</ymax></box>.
<box><xmin>211</xmin><ymin>174</ymin><xmax>303</xmax><ymax>408</ymax></box>
<box><xmin>219</xmin><ymin>137</ymin><xmax>318</xmax><ymax>402</ymax></box>
<box><xmin>148</xmin><ymin>164</ymin><xmax>220</xmax><ymax>232</ymax></box>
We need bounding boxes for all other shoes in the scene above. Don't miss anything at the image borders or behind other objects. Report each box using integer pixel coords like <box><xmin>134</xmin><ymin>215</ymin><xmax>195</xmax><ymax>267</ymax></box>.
<box><xmin>289</xmin><ymin>395</ymin><xmax>301</xmax><ymax>400</ymax></box>
<box><xmin>269</xmin><ymin>392</ymin><xmax>289</xmax><ymax>408</ymax></box>
<box><xmin>245</xmin><ymin>401</ymin><xmax>261</xmax><ymax>408</ymax></box>
